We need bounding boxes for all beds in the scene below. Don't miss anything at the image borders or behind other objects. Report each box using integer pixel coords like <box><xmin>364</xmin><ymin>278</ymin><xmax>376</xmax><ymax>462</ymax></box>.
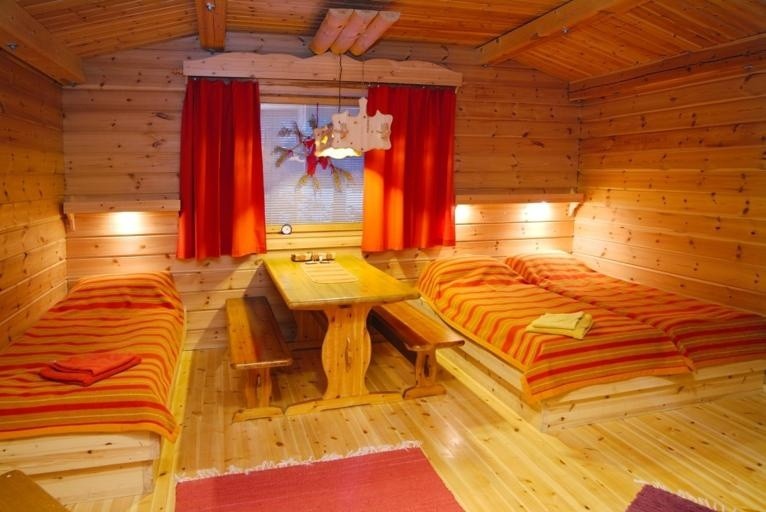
<box><xmin>414</xmin><ymin>252</ymin><xmax>766</xmax><ymax>433</ymax></box>
<box><xmin>0</xmin><ymin>270</ymin><xmax>189</xmax><ymax>503</ymax></box>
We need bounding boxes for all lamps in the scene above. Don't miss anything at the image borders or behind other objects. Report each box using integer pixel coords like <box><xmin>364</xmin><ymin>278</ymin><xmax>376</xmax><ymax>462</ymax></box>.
<box><xmin>309</xmin><ymin>43</ymin><xmax>395</xmax><ymax>161</ymax></box>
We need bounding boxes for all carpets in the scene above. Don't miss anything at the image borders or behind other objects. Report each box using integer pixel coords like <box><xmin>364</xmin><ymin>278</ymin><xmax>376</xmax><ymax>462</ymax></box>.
<box><xmin>625</xmin><ymin>482</ymin><xmax>720</xmax><ymax>512</ymax></box>
<box><xmin>172</xmin><ymin>443</ymin><xmax>465</xmax><ymax>512</ymax></box>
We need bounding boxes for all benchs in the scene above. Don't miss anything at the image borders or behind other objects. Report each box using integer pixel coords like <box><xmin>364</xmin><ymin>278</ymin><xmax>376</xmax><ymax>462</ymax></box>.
<box><xmin>371</xmin><ymin>299</ymin><xmax>465</xmax><ymax>402</ymax></box>
<box><xmin>223</xmin><ymin>294</ymin><xmax>294</xmax><ymax>423</ymax></box>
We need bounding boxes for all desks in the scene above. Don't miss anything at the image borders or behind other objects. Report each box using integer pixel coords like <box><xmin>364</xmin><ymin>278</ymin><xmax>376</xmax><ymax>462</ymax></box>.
<box><xmin>262</xmin><ymin>250</ymin><xmax>422</xmax><ymax>417</ymax></box>
